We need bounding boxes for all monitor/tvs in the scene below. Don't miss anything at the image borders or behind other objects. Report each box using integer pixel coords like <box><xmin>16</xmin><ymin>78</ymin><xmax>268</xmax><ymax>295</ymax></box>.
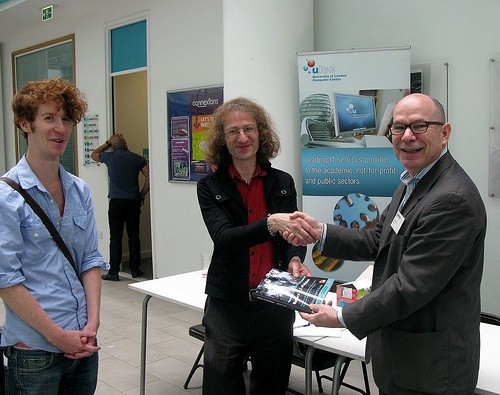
<box><xmin>331</xmin><ymin>91</ymin><xmax>377</xmax><ymax>138</ymax></box>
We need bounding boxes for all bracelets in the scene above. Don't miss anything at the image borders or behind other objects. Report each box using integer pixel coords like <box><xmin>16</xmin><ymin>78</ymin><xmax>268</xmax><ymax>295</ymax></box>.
<box><xmin>267</xmin><ymin>213</ymin><xmax>277</xmax><ymax>237</ymax></box>
<box><xmin>106</xmin><ymin>140</ymin><xmax>112</xmax><ymax>147</ymax></box>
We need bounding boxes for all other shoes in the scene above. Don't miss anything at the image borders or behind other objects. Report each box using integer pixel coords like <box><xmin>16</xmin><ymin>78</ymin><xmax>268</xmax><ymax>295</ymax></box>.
<box><xmin>133</xmin><ymin>271</ymin><xmax>143</xmax><ymax>278</ymax></box>
<box><xmin>101</xmin><ymin>274</ymin><xmax>119</xmax><ymax>281</ymax></box>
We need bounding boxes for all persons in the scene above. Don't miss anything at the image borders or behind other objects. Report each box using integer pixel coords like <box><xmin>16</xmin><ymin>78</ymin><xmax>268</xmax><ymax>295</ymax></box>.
<box><xmin>197</xmin><ymin>97</ymin><xmax>322</xmax><ymax>395</ymax></box>
<box><xmin>0</xmin><ymin>78</ymin><xmax>109</xmax><ymax>395</ymax></box>
<box><xmin>333</xmin><ymin>193</ymin><xmax>380</xmax><ymax>229</ymax></box>
<box><xmin>264</xmin><ymin>284</ymin><xmax>323</xmax><ymax>306</ymax></box>
<box><xmin>279</xmin><ymin>94</ymin><xmax>487</xmax><ymax>395</ymax></box>
<box><xmin>91</xmin><ymin>134</ymin><xmax>149</xmax><ymax>281</ymax></box>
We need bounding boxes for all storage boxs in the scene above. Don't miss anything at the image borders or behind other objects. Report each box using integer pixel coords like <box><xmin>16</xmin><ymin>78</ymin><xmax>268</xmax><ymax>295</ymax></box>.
<box><xmin>337</xmin><ymin>281</ymin><xmax>371</xmax><ymax>308</ymax></box>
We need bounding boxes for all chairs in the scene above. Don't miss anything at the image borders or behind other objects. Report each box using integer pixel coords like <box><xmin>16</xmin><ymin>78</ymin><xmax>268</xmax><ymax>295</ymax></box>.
<box><xmin>184</xmin><ymin>324</ymin><xmax>371</xmax><ymax>395</ymax></box>
<box><xmin>377</xmin><ymin>103</ymin><xmax>396</xmax><ymax>136</ymax></box>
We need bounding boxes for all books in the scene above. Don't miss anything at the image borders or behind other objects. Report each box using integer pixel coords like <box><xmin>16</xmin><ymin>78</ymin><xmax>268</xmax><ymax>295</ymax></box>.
<box><xmin>251</xmin><ymin>269</ymin><xmax>335</xmax><ymax>314</ymax></box>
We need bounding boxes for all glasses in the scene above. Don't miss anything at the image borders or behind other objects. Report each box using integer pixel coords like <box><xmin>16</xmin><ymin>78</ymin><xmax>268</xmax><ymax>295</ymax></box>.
<box><xmin>387</xmin><ymin>122</ymin><xmax>443</xmax><ymax>134</ymax></box>
<box><xmin>222</xmin><ymin>126</ymin><xmax>257</xmax><ymax>136</ymax></box>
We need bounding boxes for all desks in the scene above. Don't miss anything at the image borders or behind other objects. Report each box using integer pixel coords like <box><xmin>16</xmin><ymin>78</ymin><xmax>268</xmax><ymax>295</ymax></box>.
<box><xmin>127</xmin><ymin>269</ymin><xmax>371</xmax><ymax>395</ymax></box>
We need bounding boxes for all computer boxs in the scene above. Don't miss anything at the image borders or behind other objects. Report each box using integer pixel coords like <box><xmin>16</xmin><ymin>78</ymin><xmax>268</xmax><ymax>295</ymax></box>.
<box><xmin>360</xmin><ymin>88</ymin><xmax>409</xmax><ymax>134</ymax></box>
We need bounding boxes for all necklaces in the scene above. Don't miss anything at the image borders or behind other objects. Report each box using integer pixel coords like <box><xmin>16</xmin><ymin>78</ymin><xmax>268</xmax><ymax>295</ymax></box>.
<box><xmin>245</xmin><ymin>176</ymin><xmax>252</xmax><ymax>183</ymax></box>
